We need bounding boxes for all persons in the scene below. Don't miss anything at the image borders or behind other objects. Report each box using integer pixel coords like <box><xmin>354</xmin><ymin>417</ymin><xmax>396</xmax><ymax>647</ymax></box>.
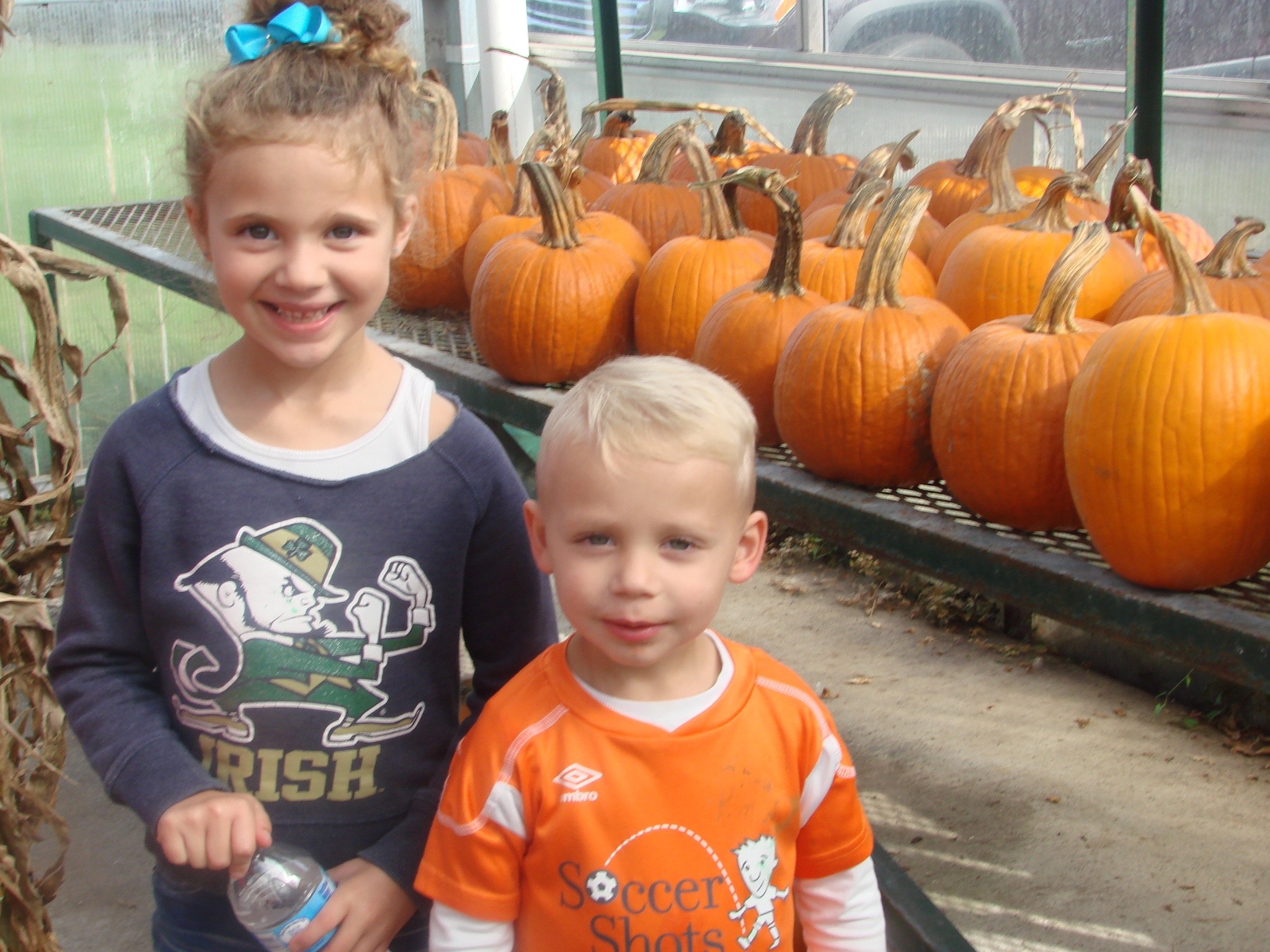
<box><xmin>48</xmin><ymin>0</ymin><xmax>560</xmax><ymax>952</ymax></box>
<box><xmin>415</xmin><ymin>354</ymin><xmax>889</xmax><ymax>951</ymax></box>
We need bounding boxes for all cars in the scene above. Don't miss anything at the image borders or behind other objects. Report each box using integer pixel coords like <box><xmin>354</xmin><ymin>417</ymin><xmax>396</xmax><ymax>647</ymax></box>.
<box><xmin>532</xmin><ymin>0</ymin><xmax>1270</xmax><ymax>85</ymax></box>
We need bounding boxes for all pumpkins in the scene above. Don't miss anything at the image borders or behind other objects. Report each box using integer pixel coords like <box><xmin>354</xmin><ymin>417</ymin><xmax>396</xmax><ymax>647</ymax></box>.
<box><xmin>387</xmin><ymin>79</ymin><xmax>1270</xmax><ymax>590</ymax></box>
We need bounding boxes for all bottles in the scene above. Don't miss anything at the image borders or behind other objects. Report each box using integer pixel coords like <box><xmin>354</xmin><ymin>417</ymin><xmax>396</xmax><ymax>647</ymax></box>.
<box><xmin>227</xmin><ymin>843</ymin><xmax>337</xmax><ymax>952</ymax></box>
